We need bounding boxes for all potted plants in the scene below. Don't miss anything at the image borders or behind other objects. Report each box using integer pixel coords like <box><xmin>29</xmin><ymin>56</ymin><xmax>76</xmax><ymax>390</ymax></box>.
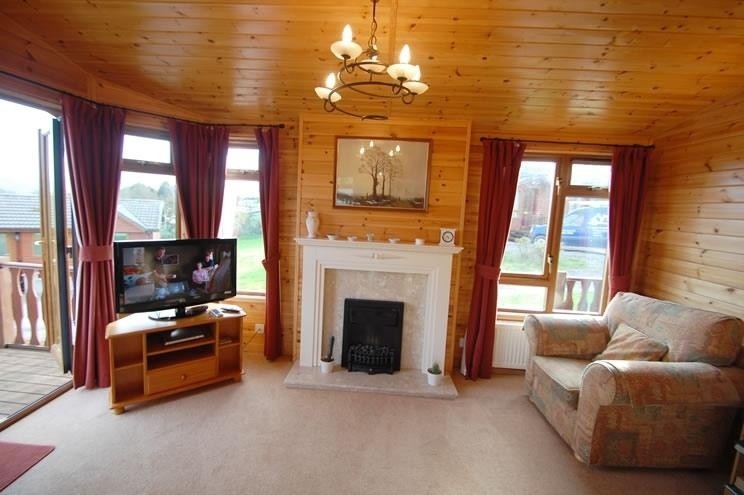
<box><xmin>320</xmin><ymin>336</ymin><xmax>335</xmax><ymax>374</ymax></box>
<box><xmin>425</xmin><ymin>363</ymin><xmax>442</xmax><ymax>386</ymax></box>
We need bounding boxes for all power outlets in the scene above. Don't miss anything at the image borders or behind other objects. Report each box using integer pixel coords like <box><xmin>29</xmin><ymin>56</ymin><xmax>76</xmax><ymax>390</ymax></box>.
<box><xmin>254</xmin><ymin>324</ymin><xmax>264</xmax><ymax>334</ymax></box>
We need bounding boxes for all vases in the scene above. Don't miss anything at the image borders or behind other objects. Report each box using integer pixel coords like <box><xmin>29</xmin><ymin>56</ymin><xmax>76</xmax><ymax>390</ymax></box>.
<box><xmin>305</xmin><ymin>211</ymin><xmax>320</xmax><ymax>238</ymax></box>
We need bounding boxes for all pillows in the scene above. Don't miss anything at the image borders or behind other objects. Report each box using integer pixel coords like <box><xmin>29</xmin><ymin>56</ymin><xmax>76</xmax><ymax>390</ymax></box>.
<box><xmin>592</xmin><ymin>321</ymin><xmax>669</xmax><ymax>363</ymax></box>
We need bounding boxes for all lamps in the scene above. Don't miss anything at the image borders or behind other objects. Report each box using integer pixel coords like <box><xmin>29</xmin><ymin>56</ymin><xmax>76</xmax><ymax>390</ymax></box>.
<box><xmin>315</xmin><ymin>0</ymin><xmax>429</xmax><ymax>121</ymax></box>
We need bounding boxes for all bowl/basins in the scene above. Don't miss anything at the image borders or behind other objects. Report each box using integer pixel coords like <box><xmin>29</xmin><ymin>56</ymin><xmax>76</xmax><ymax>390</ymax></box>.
<box><xmin>348</xmin><ymin>236</ymin><xmax>358</xmax><ymax>241</ymax></box>
<box><xmin>388</xmin><ymin>239</ymin><xmax>400</xmax><ymax>243</ymax></box>
<box><xmin>326</xmin><ymin>234</ymin><xmax>337</xmax><ymax>241</ymax></box>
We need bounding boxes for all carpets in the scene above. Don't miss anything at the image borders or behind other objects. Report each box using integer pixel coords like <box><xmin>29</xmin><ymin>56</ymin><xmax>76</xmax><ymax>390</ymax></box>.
<box><xmin>0</xmin><ymin>441</ymin><xmax>56</xmax><ymax>491</ymax></box>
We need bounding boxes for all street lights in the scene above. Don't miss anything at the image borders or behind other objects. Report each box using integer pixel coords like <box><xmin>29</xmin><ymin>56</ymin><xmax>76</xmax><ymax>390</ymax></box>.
<box><xmin>521</xmin><ymin>178</ymin><xmax>537</xmax><ymax>225</ymax></box>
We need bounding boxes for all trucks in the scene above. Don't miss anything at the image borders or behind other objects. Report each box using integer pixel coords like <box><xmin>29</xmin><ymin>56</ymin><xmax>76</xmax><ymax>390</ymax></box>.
<box><xmin>530</xmin><ymin>206</ymin><xmax>607</xmax><ymax>250</ymax></box>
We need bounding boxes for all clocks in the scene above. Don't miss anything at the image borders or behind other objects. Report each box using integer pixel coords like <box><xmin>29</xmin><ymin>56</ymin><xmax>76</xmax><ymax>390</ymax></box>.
<box><xmin>440</xmin><ymin>228</ymin><xmax>456</xmax><ymax>247</ymax></box>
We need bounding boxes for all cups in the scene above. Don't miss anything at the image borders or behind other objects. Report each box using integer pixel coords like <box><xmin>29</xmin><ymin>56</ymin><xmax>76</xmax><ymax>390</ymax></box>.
<box><xmin>415</xmin><ymin>238</ymin><xmax>424</xmax><ymax>246</ymax></box>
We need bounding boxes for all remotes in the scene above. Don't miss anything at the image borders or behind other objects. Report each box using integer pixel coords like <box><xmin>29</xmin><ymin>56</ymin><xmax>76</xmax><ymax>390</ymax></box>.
<box><xmin>221</xmin><ymin>307</ymin><xmax>240</xmax><ymax>313</ymax></box>
<box><xmin>209</xmin><ymin>307</ymin><xmax>224</xmax><ymax>318</ymax></box>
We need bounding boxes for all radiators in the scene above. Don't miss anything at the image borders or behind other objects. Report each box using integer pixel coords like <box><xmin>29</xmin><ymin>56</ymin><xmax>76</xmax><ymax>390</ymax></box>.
<box><xmin>491</xmin><ymin>320</ymin><xmax>528</xmax><ymax>370</ymax></box>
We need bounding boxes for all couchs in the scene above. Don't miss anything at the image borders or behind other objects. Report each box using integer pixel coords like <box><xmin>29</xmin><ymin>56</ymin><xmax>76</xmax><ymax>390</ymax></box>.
<box><xmin>521</xmin><ymin>291</ymin><xmax>744</xmax><ymax>470</ymax></box>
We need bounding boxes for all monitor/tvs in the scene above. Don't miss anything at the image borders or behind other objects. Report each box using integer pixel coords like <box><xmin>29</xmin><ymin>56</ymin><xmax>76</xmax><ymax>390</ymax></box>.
<box><xmin>111</xmin><ymin>235</ymin><xmax>239</xmax><ymax>319</ymax></box>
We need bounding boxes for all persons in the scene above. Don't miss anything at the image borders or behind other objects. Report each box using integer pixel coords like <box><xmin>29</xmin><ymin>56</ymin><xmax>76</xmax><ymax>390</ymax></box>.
<box><xmin>190</xmin><ymin>250</ymin><xmax>218</xmax><ymax>294</ymax></box>
<box><xmin>150</xmin><ymin>246</ymin><xmax>176</xmax><ymax>300</ymax></box>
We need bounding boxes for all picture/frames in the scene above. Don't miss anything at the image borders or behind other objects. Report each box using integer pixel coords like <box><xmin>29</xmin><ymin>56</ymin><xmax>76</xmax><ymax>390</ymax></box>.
<box><xmin>332</xmin><ymin>135</ymin><xmax>432</xmax><ymax>213</ymax></box>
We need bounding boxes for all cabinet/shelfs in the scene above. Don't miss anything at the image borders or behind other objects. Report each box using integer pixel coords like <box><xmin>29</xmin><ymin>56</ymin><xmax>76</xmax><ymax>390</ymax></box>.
<box><xmin>106</xmin><ymin>302</ymin><xmax>247</xmax><ymax>415</ymax></box>
